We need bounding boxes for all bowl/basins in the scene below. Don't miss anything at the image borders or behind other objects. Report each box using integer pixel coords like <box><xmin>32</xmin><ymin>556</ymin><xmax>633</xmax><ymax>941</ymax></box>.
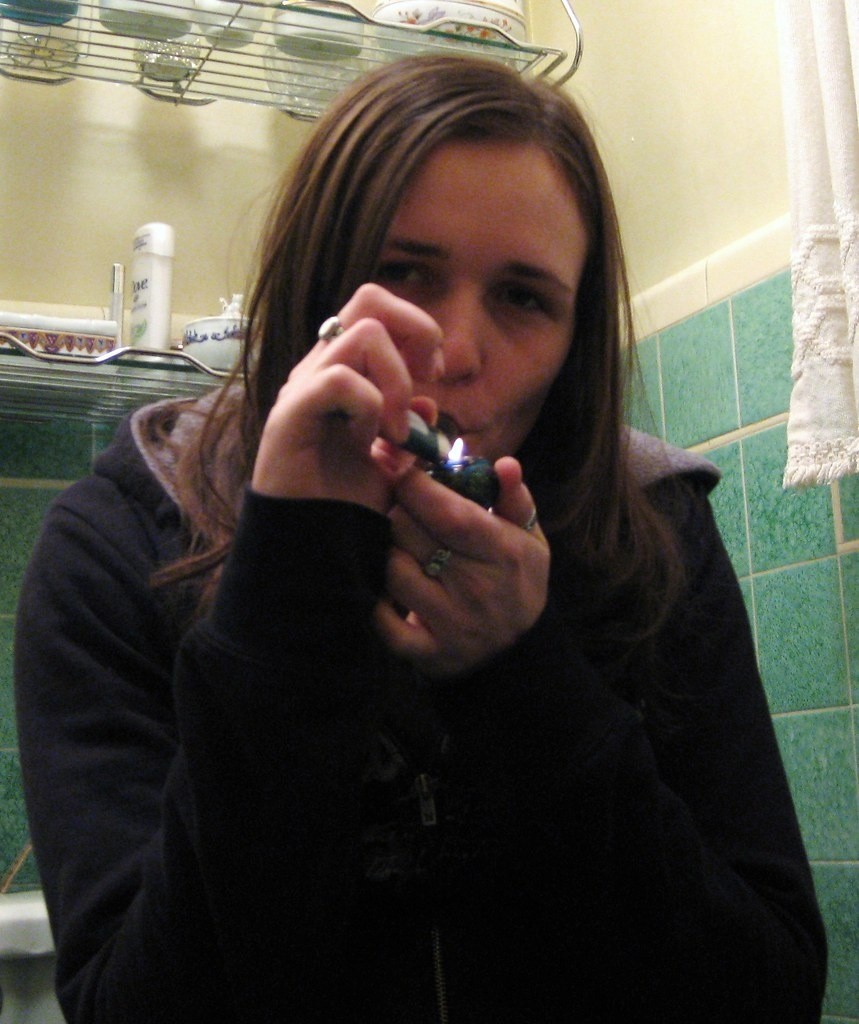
<box><xmin>196</xmin><ymin>0</ymin><xmax>265</xmax><ymax>47</ymax></box>
<box><xmin>97</xmin><ymin>0</ymin><xmax>195</xmax><ymax>39</ymax></box>
<box><xmin>182</xmin><ymin>317</ymin><xmax>264</xmax><ymax>373</ymax></box>
<box><xmin>271</xmin><ymin>1</ymin><xmax>364</xmax><ymax>61</ymax></box>
<box><xmin>0</xmin><ymin>0</ymin><xmax>79</xmax><ymax>27</ymax></box>
<box><xmin>263</xmin><ymin>45</ymin><xmax>373</xmax><ymax>123</ymax></box>
<box><xmin>134</xmin><ymin>37</ymin><xmax>200</xmax><ymax>82</ymax></box>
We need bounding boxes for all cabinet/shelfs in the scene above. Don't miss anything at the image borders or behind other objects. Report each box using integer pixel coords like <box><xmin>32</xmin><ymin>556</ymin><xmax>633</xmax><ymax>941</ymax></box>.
<box><xmin>0</xmin><ymin>0</ymin><xmax>584</xmax><ymax>428</ymax></box>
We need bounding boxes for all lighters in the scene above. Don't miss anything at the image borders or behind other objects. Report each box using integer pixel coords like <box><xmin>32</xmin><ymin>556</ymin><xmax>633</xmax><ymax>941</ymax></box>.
<box><xmin>378</xmin><ymin>408</ymin><xmax>502</xmax><ymax>516</ymax></box>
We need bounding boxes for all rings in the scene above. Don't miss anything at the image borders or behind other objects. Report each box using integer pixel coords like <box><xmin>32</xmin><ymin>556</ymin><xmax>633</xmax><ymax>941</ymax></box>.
<box><xmin>422</xmin><ymin>541</ymin><xmax>453</xmax><ymax>577</ymax></box>
<box><xmin>317</xmin><ymin>315</ymin><xmax>345</xmax><ymax>340</ymax></box>
<box><xmin>520</xmin><ymin>505</ymin><xmax>538</xmax><ymax>532</ymax></box>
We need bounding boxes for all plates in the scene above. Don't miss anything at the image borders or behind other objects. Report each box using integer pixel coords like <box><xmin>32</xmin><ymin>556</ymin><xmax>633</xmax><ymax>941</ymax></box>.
<box><xmin>371</xmin><ymin>0</ymin><xmax>526</xmax><ymax>62</ymax></box>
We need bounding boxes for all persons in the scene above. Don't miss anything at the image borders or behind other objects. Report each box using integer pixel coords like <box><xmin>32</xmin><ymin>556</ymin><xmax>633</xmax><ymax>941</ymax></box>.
<box><xmin>14</xmin><ymin>53</ymin><xmax>828</xmax><ymax>1024</ymax></box>
